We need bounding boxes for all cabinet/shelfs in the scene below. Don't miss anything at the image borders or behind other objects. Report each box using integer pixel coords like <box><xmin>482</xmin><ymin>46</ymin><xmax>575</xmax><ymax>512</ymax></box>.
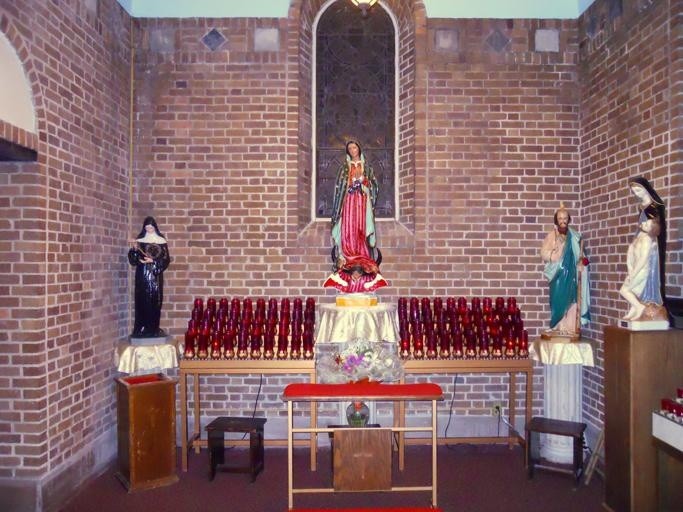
<box><xmin>603</xmin><ymin>324</ymin><xmax>683</xmax><ymax>512</ymax></box>
<box><xmin>180</xmin><ymin>302</ymin><xmax>534</xmax><ymax>474</ymax></box>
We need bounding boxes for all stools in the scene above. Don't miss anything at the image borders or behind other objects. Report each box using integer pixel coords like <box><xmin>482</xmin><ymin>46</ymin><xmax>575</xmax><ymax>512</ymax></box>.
<box><xmin>524</xmin><ymin>416</ymin><xmax>586</xmax><ymax>487</ymax></box>
<box><xmin>204</xmin><ymin>417</ymin><xmax>266</xmax><ymax>483</ymax></box>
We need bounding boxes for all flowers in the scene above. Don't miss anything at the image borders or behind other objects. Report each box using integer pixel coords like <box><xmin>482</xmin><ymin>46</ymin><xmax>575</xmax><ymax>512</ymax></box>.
<box><xmin>336</xmin><ymin>338</ymin><xmax>405</xmax><ymax>383</ymax></box>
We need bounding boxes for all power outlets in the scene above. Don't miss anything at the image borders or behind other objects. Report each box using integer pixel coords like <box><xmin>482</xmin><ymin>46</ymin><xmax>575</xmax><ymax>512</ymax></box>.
<box><xmin>493</xmin><ymin>403</ymin><xmax>502</xmax><ymax>416</ymax></box>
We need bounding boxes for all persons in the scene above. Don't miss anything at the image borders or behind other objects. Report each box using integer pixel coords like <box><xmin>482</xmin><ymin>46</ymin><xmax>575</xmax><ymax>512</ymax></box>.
<box><xmin>540</xmin><ymin>209</ymin><xmax>590</xmax><ymax>341</ymax></box>
<box><xmin>323</xmin><ymin>138</ymin><xmax>389</xmax><ymax>292</ymax></box>
<box><xmin>620</xmin><ymin>176</ymin><xmax>667</xmax><ymax>320</ymax></box>
<box><xmin>128</xmin><ymin>217</ymin><xmax>171</xmax><ymax>336</ymax></box>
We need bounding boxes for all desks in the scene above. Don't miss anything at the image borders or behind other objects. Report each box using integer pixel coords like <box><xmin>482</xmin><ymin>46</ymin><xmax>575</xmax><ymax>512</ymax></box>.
<box><xmin>280</xmin><ymin>383</ymin><xmax>444</xmax><ymax>509</ymax></box>
